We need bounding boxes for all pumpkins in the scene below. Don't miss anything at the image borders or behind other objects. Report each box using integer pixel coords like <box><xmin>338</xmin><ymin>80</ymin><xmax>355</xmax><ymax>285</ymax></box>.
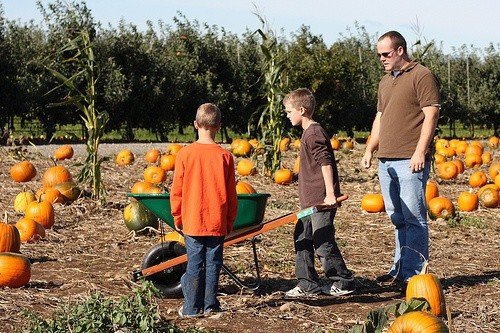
<box><xmin>425</xmin><ymin>136</ymin><xmax>500</xmax><ymax>220</ymax></box>
<box><xmin>54</xmin><ymin>144</ymin><xmax>74</xmax><ymax>160</ymax></box>
<box><xmin>116</xmin><ymin>135</ymin><xmax>353</xmax><ymax>243</ymax></box>
<box><xmin>0</xmin><ymin>161</ymin><xmax>81</xmax><ymax>288</ymax></box>
<box><xmin>387</xmin><ymin>310</ymin><xmax>449</xmax><ymax>333</ymax></box>
<box><xmin>406</xmin><ymin>262</ymin><xmax>446</xmax><ymax>319</ymax></box>
<box><xmin>361</xmin><ymin>192</ymin><xmax>385</xmax><ymax>212</ymax></box>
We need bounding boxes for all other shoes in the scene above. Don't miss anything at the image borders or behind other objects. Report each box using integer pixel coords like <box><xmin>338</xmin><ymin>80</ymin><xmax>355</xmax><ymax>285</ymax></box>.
<box><xmin>389</xmin><ymin>279</ymin><xmax>409</xmax><ymax>293</ymax></box>
<box><xmin>376</xmin><ymin>274</ymin><xmax>394</xmax><ymax>282</ymax></box>
<box><xmin>178</xmin><ymin>306</ymin><xmax>200</xmax><ymax>318</ymax></box>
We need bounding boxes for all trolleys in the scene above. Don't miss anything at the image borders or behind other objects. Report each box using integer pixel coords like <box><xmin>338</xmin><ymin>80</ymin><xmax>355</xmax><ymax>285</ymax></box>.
<box><xmin>126</xmin><ymin>192</ymin><xmax>350</xmax><ymax>298</ymax></box>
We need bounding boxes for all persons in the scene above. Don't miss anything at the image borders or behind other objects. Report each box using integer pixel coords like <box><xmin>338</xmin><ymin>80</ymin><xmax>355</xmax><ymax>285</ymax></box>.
<box><xmin>170</xmin><ymin>104</ymin><xmax>237</xmax><ymax>318</ymax></box>
<box><xmin>362</xmin><ymin>31</ymin><xmax>442</xmax><ymax>289</ymax></box>
<box><xmin>283</xmin><ymin>88</ymin><xmax>357</xmax><ymax>298</ymax></box>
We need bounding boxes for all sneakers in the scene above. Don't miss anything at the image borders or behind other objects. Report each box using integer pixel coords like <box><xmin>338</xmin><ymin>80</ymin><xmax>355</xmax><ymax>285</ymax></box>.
<box><xmin>285</xmin><ymin>286</ymin><xmax>322</xmax><ymax>297</ymax></box>
<box><xmin>323</xmin><ymin>283</ymin><xmax>356</xmax><ymax>296</ymax></box>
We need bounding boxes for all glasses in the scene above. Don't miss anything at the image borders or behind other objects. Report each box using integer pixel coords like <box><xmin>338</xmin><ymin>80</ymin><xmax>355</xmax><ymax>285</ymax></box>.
<box><xmin>377</xmin><ymin>47</ymin><xmax>399</xmax><ymax>58</ymax></box>
